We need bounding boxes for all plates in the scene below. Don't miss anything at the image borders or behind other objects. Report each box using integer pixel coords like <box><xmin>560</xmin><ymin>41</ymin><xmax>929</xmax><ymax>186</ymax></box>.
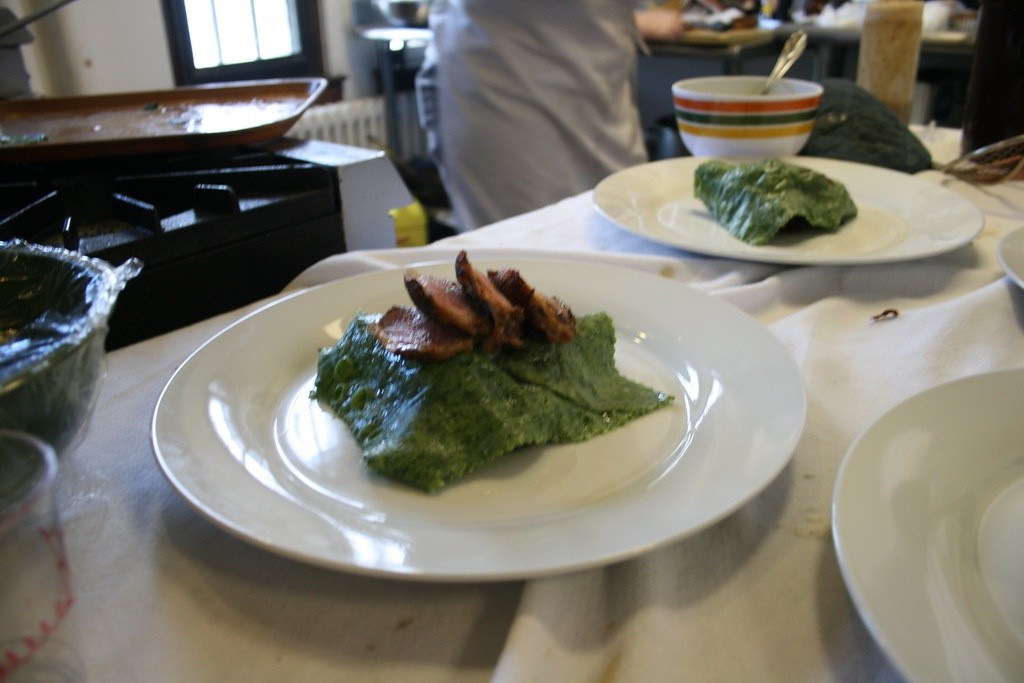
<box><xmin>149</xmin><ymin>259</ymin><xmax>806</xmax><ymax>583</ymax></box>
<box><xmin>591</xmin><ymin>155</ymin><xmax>984</xmax><ymax>265</ymax></box>
<box><xmin>832</xmin><ymin>369</ymin><xmax>1024</xmax><ymax>683</ymax></box>
<box><xmin>995</xmin><ymin>226</ymin><xmax>1024</xmax><ymax>291</ymax></box>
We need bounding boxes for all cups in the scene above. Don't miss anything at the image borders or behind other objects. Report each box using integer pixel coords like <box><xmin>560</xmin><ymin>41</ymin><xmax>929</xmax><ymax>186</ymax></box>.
<box><xmin>0</xmin><ymin>430</ymin><xmax>86</xmax><ymax>683</ymax></box>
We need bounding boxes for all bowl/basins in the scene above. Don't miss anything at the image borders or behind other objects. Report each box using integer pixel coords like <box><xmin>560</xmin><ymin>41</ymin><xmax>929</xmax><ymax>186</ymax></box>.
<box><xmin>670</xmin><ymin>74</ymin><xmax>825</xmax><ymax>155</ymax></box>
<box><xmin>0</xmin><ymin>241</ymin><xmax>119</xmax><ymax>463</ymax></box>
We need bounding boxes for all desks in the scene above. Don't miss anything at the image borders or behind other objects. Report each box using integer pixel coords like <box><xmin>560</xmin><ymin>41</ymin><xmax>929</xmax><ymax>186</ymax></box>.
<box><xmin>0</xmin><ymin>128</ymin><xmax>1024</xmax><ymax>683</ymax></box>
<box><xmin>354</xmin><ymin>20</ymin><xmax>981</xmax><ymax>168</ymax></box>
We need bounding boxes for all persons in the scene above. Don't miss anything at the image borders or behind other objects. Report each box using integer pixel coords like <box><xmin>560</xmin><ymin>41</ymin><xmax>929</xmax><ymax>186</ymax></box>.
<box><xmin>961</xmin><ymin>0</ymin><xmax>1024</xmax><ymax>157</ymax></box>
<box><xmin>413</xmin><ymin>0</ymin><xmax>649</xmax><ymax>233</ymax></box>
